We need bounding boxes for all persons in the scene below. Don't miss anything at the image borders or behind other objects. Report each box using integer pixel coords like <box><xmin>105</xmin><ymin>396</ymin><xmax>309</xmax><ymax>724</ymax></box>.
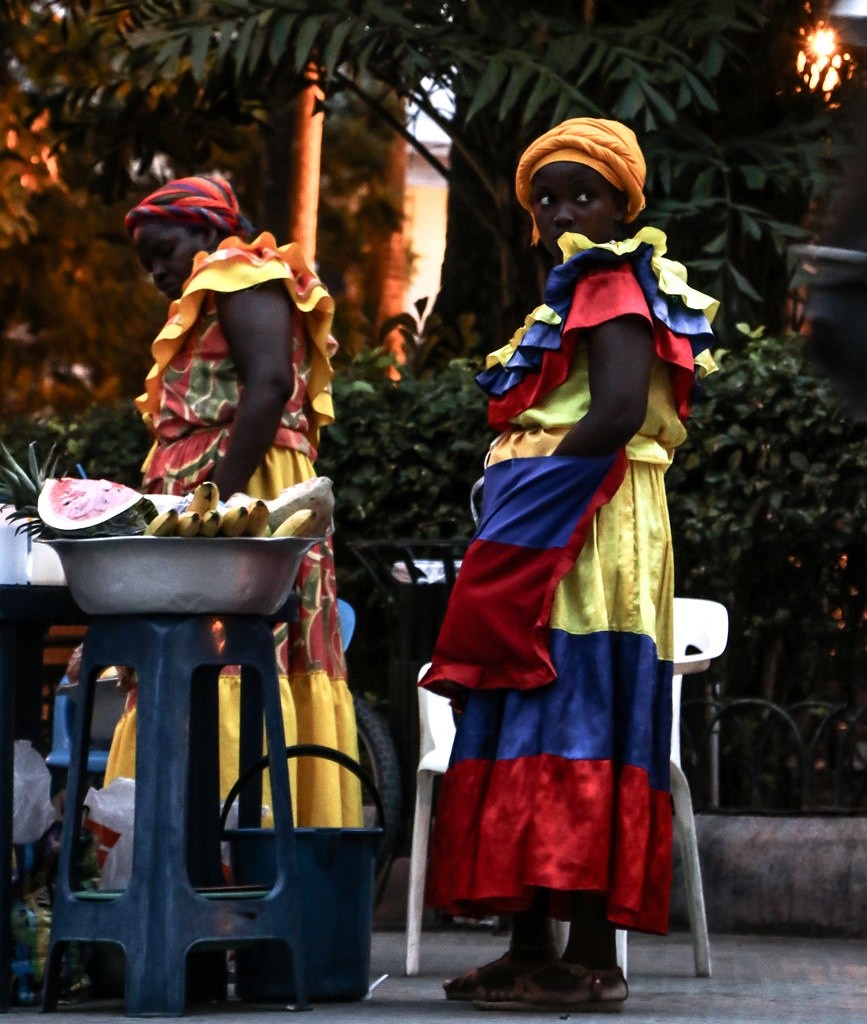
<box><xmin>98</xmin><ymin>178</ymin><xmax>363</xmax><ymax>827</ymax></box>
<box><xmin>415</xmin><ymin>117</ymin><xmax>721</xmax><ymax>1010</ymax></box>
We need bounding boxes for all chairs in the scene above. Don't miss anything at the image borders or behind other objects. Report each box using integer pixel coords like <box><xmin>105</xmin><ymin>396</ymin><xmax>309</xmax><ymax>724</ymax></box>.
<box><xmin>400</xmin><ymin>597</ymin><xmax>729</xmax><ymax>979</ymax></box>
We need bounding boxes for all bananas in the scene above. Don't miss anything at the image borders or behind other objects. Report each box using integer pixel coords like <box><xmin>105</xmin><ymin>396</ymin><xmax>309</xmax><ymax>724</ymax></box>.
<box><xmin>142</xmin><ymin>482</ymin><xmax>317</xmax><ymax>538</ymax></box>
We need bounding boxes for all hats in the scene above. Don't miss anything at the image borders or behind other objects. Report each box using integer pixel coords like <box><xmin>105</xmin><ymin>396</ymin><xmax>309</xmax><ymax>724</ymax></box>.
<box><xmin>515</xmin><ymin>117</ymin><xmax>646</xmax><ymax>244</ymax></box>
<box><xmin>124</xmin><ymin>176</ymin><xmax>243</xmax><ymax>241</ymax></box>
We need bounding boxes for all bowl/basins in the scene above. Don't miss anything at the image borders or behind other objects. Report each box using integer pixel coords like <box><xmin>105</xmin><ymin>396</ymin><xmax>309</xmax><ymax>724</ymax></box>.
<box><xmin>30</xmin><ymin>535</ymin><xmax>324</xmax><ymax>614</ymax></box>
<box><xmin>55</xmin><ymin>675</ymin><xmax>127</xmax><ymax>741</ymax></box>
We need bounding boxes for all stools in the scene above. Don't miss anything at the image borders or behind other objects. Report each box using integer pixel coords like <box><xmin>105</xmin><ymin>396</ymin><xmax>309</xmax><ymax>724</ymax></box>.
<box><xmin>38</xmin><ymin>621</ymin><xmax>315</xmax><ymax>1018</ymax></box>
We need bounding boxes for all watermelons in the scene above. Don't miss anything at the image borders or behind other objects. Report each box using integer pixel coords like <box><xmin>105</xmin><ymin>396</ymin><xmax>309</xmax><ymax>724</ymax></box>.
<box><xmin>38</xmin><ymin>478</ymin><xmax>158</xmax><ymax>536</ymax></box>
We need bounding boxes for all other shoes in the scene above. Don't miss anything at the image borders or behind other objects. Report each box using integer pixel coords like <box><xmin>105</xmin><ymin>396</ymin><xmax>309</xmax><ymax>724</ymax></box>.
<box><xmin>445</xmin><ymin>954</ymin><xmax>590</xmax><ymax>1003</ymax></box>
<box><xmin>473</xmin><ymin>967</ymin><xmax>629</xmax><ymax>1012</ymax></box>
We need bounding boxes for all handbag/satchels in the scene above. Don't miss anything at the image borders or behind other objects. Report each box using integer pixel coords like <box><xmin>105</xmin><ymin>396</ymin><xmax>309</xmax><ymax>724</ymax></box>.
<box><xmin>85</xmin><ymin>778</ymin><xmax>271</xmax><ymax>888</ymax></box>
